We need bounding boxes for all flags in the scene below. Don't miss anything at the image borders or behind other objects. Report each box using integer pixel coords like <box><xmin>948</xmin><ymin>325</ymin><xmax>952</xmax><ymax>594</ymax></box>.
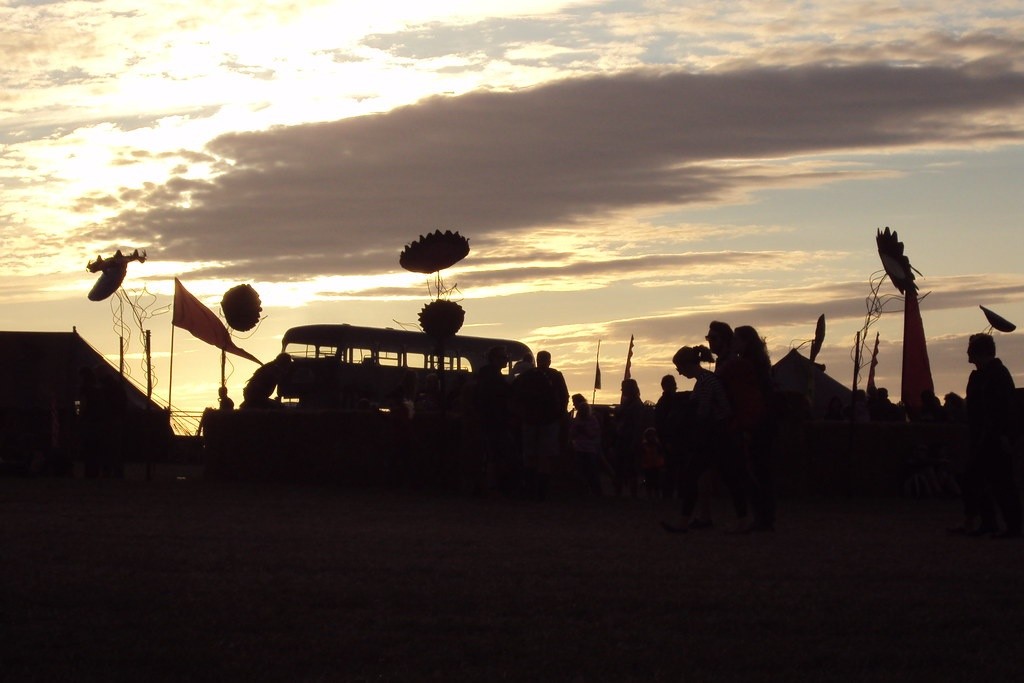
<box><xmin>173</xmin><ymin>281</ymin><xmax>264</xmax><ymax>369</ymax></box>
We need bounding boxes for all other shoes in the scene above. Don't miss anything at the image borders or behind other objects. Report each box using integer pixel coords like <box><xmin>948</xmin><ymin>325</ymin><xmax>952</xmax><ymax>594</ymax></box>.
<box><xmin>688</xmin><ymin>519</ymin><xmax>713</xmax><ymax>529</ymax></box>
<box><xmin>662</xmin><ymin>523</ymin><xmax>688</xmax><ymax>533</ymax></box>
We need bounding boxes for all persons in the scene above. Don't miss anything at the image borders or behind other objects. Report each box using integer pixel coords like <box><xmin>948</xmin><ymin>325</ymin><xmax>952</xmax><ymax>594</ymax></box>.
<box><xmin>219</xmin><ymin>388</ymin><xmax>234</xmax><ymax>410</ymax></box>
<box><xmin>371</xmin><ymin>311</ymin><xmax>965</xmax><ymax>534</ymax></box>
<box><xmin>240</xmin><ymin>353</ymin><xmax>293</xmax><ymax>409</ymax></box>
<box><xmin>946</xmin><ymin>332</ymin><xmax>1024</xmax><ymax>540</ymax></box>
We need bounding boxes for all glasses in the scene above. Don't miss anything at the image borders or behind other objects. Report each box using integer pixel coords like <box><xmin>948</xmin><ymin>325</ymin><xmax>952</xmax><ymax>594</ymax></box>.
<box><xmin>705</xmin><ymin>335</ymin><xmax>722</xmax><ymax>341</ymax></box>
